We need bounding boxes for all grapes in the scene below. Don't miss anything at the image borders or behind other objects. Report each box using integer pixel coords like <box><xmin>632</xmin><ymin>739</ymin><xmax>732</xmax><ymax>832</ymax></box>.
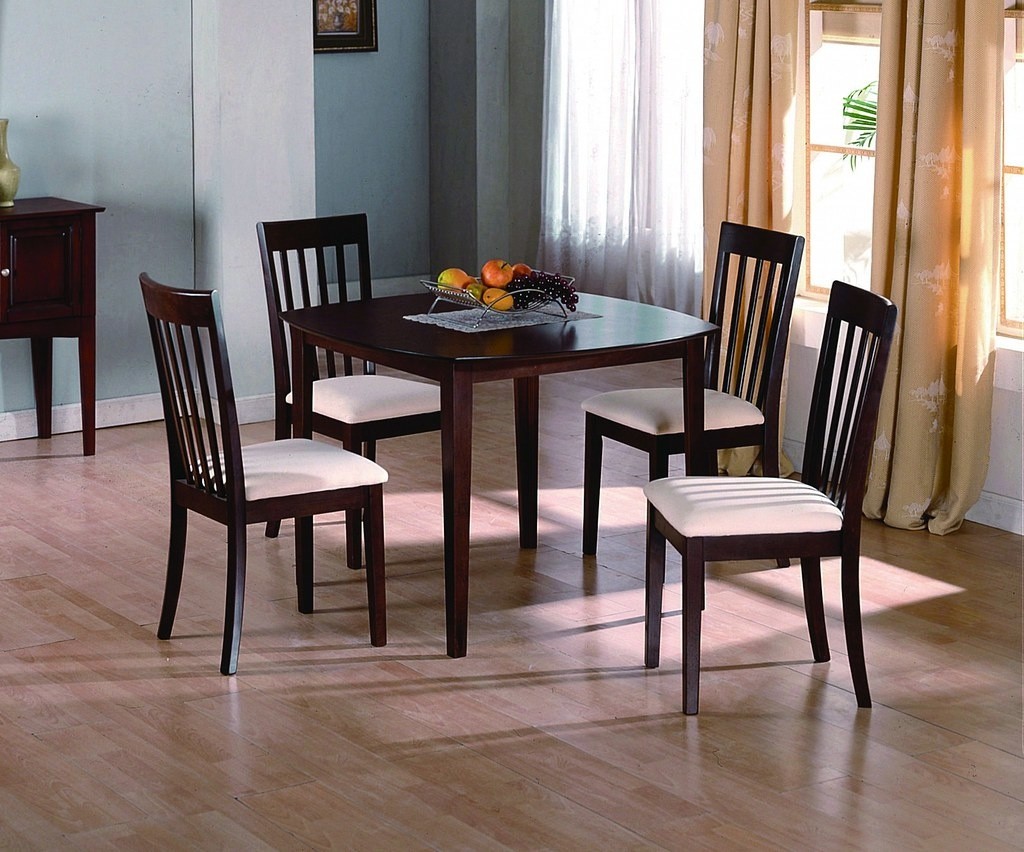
<box><xmin>506</xmin><ymin>271</ymin><xmax>579</xmax><ymax>311</ymax></box>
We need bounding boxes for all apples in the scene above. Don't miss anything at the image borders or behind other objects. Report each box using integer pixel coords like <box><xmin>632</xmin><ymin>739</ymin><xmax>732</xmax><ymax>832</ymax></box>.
<box><xmin>438</xmin><ymin>268</ymin><xmax>478</xmax><ymax>294</ymax></box>
<box><xmin>481</xmin><ymin>259</ymin><xmax>532</xmax><ymax>288</ymax></box>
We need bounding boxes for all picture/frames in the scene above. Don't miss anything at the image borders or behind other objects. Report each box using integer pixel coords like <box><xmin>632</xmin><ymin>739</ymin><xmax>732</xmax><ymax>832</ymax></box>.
<box><xmin>313</xmin><ymin>0</ymin><xmax>378</xmax><ymax>52</ymax></box>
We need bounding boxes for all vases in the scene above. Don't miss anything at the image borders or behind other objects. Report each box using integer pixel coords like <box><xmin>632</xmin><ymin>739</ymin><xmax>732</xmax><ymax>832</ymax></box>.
<box><xmin>0</xmin><ymin>119</ymin><xmax>21</xmax><ymax>208</ymax></box>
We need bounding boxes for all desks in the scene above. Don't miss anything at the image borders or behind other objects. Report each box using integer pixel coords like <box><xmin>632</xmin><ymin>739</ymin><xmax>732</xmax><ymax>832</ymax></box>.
<box><xmin>0</xmin><ymin>197</ymin><xmax>106</xmax><ymax>456</ymax></box>
<box><xmin>279</xmin><ymin>290</ymin><xmax>723</xmax><ymax>658</ymax></box>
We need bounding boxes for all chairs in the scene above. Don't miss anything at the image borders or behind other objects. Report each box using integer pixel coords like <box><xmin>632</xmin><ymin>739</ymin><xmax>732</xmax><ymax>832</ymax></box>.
<box><xmin>256</xmin><ymin>212</ymin><xmax>441</xmax><ymax>571</ymax></box>
<box><xmin>643</xmin><ymin>280</ymin><xmax>897</xmax><ymax>716</ymax></box>
<box><xmin>138</xmin><ymin>271</ymin><xmax>389</xmax><ymax>675</ymax></box>
<box><xmin>578</xmin><ymin>221</ymin><xmax>805</xmax><ymax>555</ymax></box>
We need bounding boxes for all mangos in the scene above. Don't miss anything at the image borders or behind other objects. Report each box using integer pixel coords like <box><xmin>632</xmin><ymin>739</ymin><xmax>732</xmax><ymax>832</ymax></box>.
<box><xmin>465</xmin><ymin>283</ymin><xmax>514</xmax><ymax>310</ymax></box>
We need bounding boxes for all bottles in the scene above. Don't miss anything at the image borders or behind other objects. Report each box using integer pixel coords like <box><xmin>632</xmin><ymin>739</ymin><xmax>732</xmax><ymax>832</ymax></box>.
<box><xmin>0</xmin><ymin>117</ymin><xmax>21</xmax><ymax>208</ymax></box>
